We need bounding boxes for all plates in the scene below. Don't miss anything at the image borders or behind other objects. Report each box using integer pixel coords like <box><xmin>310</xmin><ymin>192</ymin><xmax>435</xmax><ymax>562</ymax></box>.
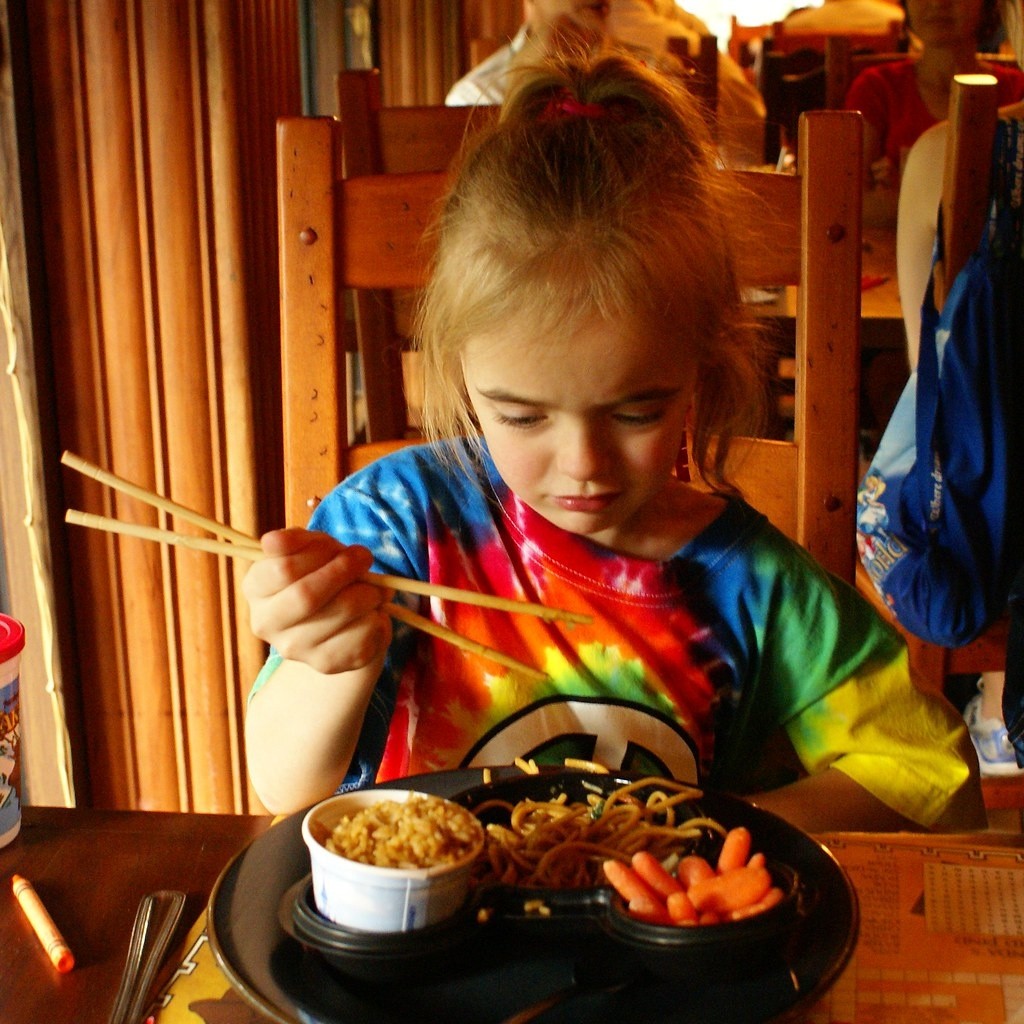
<box><xmin>207</xmin><ymin>765</ymin><xmax>859</xmax><ymax>1024</ymax></box>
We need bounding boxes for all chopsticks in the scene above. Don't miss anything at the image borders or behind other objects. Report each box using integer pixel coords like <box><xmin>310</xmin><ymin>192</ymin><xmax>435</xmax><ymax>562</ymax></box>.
<box><xmin>61</xmin><ymin>451</ymin><xmax>592</xmax><ymax>681</ymax></box>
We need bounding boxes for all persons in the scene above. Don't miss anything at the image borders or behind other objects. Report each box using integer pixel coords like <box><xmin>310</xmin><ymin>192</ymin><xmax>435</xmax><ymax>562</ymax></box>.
<box><xmin>786</xmin><ymin>0</ymin><xmax>904</xmax><ymax>33</ymax></box>
<box><xmin>896</xmin><ymin>0</ymin><xmax>1024</xmax><ymax>776</ymax></box>
<box><xmin>846</xmin><ymin>0</ymin><xmax>1024</xmax><ymax>227</ymax></box>
<box><xmin>445</xmin><ymin>0</ymin><xmax>768</xmax><ymax>123</ymax></box>
<box><xmin>243</xmin><ymin>52</ymin><xmax>989</xmax><ymax>832</ymax></box>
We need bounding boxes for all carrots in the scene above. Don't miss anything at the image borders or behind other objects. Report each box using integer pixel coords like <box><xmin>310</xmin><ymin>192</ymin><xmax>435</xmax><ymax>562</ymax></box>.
<box><xmin>602</xmin><ymin>825</ymin><xmax>785</xmax><ymax>927</ymax></box>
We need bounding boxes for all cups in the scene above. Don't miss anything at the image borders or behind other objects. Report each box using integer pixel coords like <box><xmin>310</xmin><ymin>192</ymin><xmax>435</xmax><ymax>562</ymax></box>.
<box><xmin>0</xmin><ymin>613</ymin><xmax>25</xmax><ymax>849</ymax></box>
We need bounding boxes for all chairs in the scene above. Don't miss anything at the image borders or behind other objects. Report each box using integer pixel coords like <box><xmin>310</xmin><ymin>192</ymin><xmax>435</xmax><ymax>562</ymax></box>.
<box><xmin>274</xmin><ymin>16</ymin><xmax>1023</xmax><ymax>780</ymax></box>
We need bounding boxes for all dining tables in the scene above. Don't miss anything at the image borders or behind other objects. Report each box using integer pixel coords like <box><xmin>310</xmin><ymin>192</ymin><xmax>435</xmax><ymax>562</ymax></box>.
<box><xmin>1</xmin><ymin>806</ymin><xmax>1023</xmax><ymax>1024</ymax></box>
<box><xmin>724</xmin><ymin>234</ymin><xmax>913</xmax><ymax>433</ymax></box>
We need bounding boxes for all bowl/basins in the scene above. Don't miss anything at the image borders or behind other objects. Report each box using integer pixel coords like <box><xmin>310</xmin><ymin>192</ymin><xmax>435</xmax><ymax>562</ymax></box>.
<box><xmin>451</xmin><ymin>772</ymin><xmax>705</xmax><ymax>922</ymax></box>
<box><xmin>609</xmin><ymin>852</ymin><xmax>802</xmax><ymax>958</ymax></box>
<box><xmin>302</xmin><ymin>789</ymin><xmax>485</xmax><ymax>932</ymax></box>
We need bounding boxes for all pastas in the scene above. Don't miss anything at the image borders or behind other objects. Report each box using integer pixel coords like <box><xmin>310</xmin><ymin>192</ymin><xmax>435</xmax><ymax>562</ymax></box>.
<box><xmin>471</xmin><ymin>758</ymin><xmax>727</xmax><ymax>891</ymax></box>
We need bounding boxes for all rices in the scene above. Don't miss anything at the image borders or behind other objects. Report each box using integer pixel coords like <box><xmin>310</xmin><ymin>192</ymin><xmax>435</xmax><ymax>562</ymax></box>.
<box><xmin>315</xmin><ymin>789</ymin><xmax>481</xmax><ymax>872</ymax></box>
